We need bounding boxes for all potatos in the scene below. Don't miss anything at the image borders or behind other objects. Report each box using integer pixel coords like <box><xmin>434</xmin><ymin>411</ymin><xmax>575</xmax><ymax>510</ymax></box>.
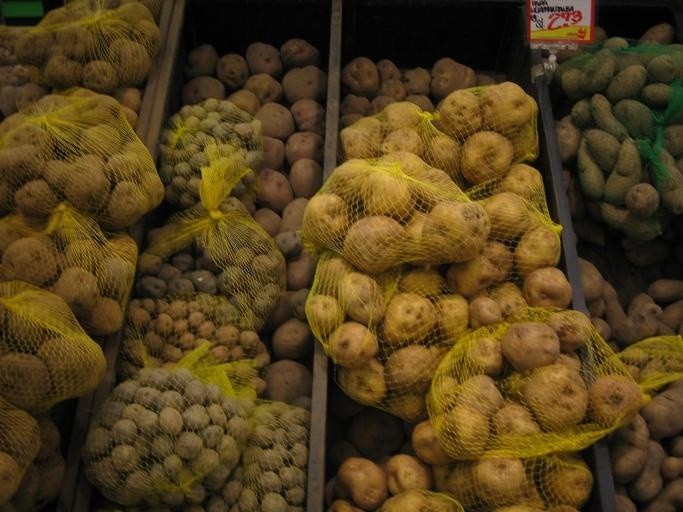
<box><xmin>0</xmin><ymin>0</ymin><xmax>683</xmax><ymax>512</ymax></box>
